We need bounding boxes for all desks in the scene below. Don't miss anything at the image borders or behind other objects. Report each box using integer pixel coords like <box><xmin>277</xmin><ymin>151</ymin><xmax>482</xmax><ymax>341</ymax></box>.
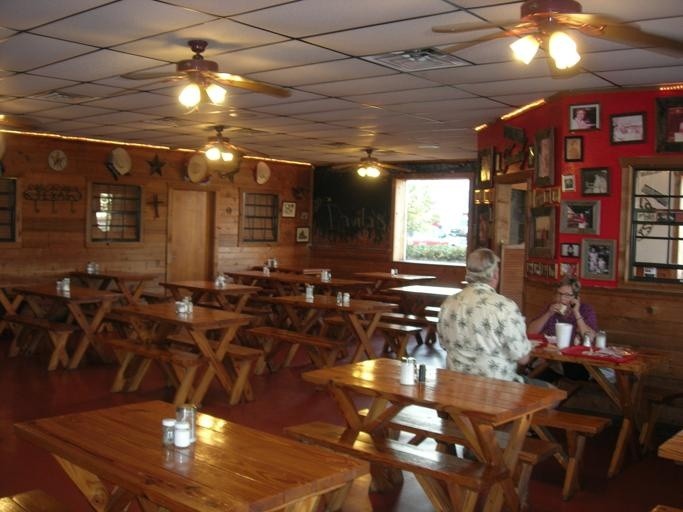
<box><xmin>69</xmin><ymin>271</ymin><xmax>158</xmax><ymax>310</ymax></box>
<box><xmin>519</xmin><ymin>344</ymin><xmax>673</xmax><ymax>479</ymax></box>
<box><xmin>352</xmin><ymin>271</ymin><xmax>437</xmax><ymax>293</ymax></box>
<box><xmin>256</xmin><ymin>294</ymin><xmax>399</xmax><ymax>367</ymax></box>
<box><xmin>301</xmin><ymin>360</ymin><xmax>567</xmax><ymax>512</ymax></box>
<box><xmin>381</xmin><ymin>285</ymin><xmax>462</xmax><ymax>345</ymax></box>
<box><xmin>224</xmin><ymin>271</ymin><xmax>376</xmax><ymax>329</ymax></box>
<box><xmin>112</xmin><ymin>301</ymin><xmax>256</xmax><ymax>408</ymax></box>
<box><xmin>13</xmin><ymin>399</ymin><xmax>370</xmax><ymax>512</ymax></box>
<box><xmin>158</xmin><ymin>280</ymin><xmax>262</xmax><ymax>347</ymax></box>
<box><xmin>0</xmin><ymin>283</ymin><xmax>125</xmax><ymax>370</ymax></box>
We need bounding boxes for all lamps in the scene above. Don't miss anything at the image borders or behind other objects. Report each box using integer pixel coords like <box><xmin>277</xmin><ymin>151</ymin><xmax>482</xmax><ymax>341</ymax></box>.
<box><xmin>177</xmin><ymin>74</ymin><xmax>226</xmax><ymax>111</ymax></box>
<box><xmin>357</xmin><ymin>166</ymin><xmax>380</xmax><ymax>177</ymax></box>
<box><xmin>509</xmin><ymin>22</ymin><xmax>581</xmax><ymax>69</ymax></box>
<box><xmin>206</xmin><ymin>145</ymin><xmax>235</xmax><ymax>162</ymax></box>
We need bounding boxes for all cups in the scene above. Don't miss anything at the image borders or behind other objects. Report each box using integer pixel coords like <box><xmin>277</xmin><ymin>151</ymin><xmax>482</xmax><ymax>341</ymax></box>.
<box><xmin>558</xmin><ymin>303</ymin><xmax>567</xmax><ymax>314</ymax></box>
<box><xmin>555</xmin><ymin>323</ymin><xmax>573</xmax><ymax>348</ymax></box>
<box><xmin>391</xmin><ymin>268</ymin><xmax>398</xmax><ymax>278</ymax></box>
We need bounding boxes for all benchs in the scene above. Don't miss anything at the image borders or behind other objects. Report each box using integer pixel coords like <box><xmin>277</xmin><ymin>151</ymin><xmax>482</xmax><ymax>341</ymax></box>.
<box><xmin>246</xmin><ymin>327</ymin><xmax>345</xmax><ymax>393</ymax></box>
<box><xmin>438</xmin><ymin>409</ymin><xmax>612</xmax><ymax>501</ymax></box>
<box><xmin>424</xmin><ymin>306</ymin><xmax>441</xmax><ymax>344</ymax></box>
<box><xmin>285</xmin><ymin>422</ymin><xmax>510</xmax><ymax>512</ymax></box>
<box><xmin>110</xmin><ymin>340</ymin><xmax>207</xmax><ymax>410</ymax></box>
<box><xmin>382</xmin><ymin>313</ymin><xmax>438</xmax><ymax>353</ymax></box>
<box><xmin>226</xmin><ymin>294</ymin><xmax>274</xmax><ymax>328</ymax></box>
<box><xmin>357</xmin><ymin>294</ymin><xmax>400</xmax><ymax>320</ymax></box>
<box><xmin>84</xmin><ymin>311</ymin><xmax>144</xmax><ymax>339</ymax></box>
<box><xmin>166</xmin><ymin>334</ymin><xmax>263</xmax><ymax>405</ymax></box>
<box><xmin>321</xmin><ymin>317</ymin><xmax>422</xmax><ymax>358</ymax></box>
<box><xmin>356</xmin><ymin>405</ymin><xmax>561</xmax><ymax>512</ymax></box>
<box><xmin>576</xmin><ymin>379</ymin><xmax>683</xmax><ymax>460</ymax></box>
<box><xmin>0</xmin><ymin>315</ymin><xmax>80</xmax><ymax>372</ymax></box>
<box><xmin>111</xmin><ymin>289</ymin><xmax>173</xmax><ymax>304</ymax></box>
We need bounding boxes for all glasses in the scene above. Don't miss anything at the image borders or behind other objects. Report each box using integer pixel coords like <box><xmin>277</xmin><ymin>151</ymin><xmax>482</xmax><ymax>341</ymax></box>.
<box><xmin>556</xmin><ymin>292</ymin><xmax>576</xmax><ymax>298</ymax></box>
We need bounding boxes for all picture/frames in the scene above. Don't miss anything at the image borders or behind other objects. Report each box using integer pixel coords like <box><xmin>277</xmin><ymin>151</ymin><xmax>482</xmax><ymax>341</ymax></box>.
<box><xmin>534</xmin><ymin>128</ymin><xmax>556</xmax><ymax>186</ymax></box>
<box><xmin>568</xmin><ymin>103</ymin><xmax>600</xmax><ymax>132</ymax></box>
<box><xmin>559</xmin><ymin>262</ymin><xmax>580</xmax><ymax>279</ymax></box>
<box><xmin>565</xmin><ymin>136</ymin><xmax>583</xmax><ymax>161</ymax></box>
<box><xmin>281</xmin><ymin>201</ymin><xmax>296</xmax><ymax>219</ymax></box>
<box><xmin>548</xmin><ymin>263</ymin><xmax>558</xmax><ymax>279</ymax></box>
<box><xmin>529</xmin><ymin>206</ymin><xmax>557</xmax><ymax>259</ymax></box>
<box><xmin>609</xmin><ymin>112</ymin><xmax>646</xmax><ymax>145</ymax></box>
<box><xmin>495</xmin><ymin>153</ymin><xmax>501</xmax><ymax>172</ymax></box>
<box><xmin>580</xmin><ymin>167</ymin><xmax>609</xmax><ymax>196</ymax></box>
<box><xmin>655</xmin><ymin>96</ymin><xmax>683</xmax><ymax>152</ymax></box>
<box><xmin>296</xmin><ymin>227</ymin><xmax>310</xmax><ymax>243</ymax></box>
<box><xmin>580</xmin><ymin>238</ymin><xmax>618</xmax><ymax>281</ymax></box>
<box><xmin>561</xmin><ymin>174</ymin><xmax>575</xmax><ymax>191</ymax></box>
<box><xmin>478</xmin><ymin>146</ymin><xmax>493</xmax><ymax>188</ymax></box>
<box><xmin>559</xmin><ymin>199</ymin><xmax>601</xmax><ymax>235</ymax></box>
<box><xmin>560</xmin><ymin>242</ymin><xmax>580</xmax><ymax>258</ymax></box>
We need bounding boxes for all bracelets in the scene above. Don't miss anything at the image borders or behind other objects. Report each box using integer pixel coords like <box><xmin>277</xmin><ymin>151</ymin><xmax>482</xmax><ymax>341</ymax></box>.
<box><xmin>575</xmin><ymin>318</ymin><xmax>585</xmax><ymax>321</ymax></box>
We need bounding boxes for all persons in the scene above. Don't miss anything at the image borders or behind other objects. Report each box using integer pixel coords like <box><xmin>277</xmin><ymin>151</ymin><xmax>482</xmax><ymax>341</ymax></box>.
<box><xmin>567</xmin><ymin>244</ymin><xmax>574</xmax><ymax>255</ymax></box>
<box><xmin>526</xmin><ymin>277</ymin><xmax>596</xmax><ymax>383</ymax></box>
<box><xmin>439</xmin><ymin>248</ymin><xmax>560</xmax><ymax>408</ymax></box>
<box><xmin>573</xmin><ymin>109</ymin><xmax>592</xmax><ymax>130</ymax></box>
<box><xmin>588</xmin><ymin>247</ymin><xmax>606</xmax><ymax>273</ymax></box>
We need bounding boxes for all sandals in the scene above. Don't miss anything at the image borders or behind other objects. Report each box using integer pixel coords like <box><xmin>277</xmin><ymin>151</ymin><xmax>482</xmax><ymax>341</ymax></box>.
<box><xmin>526</xmin><ymin>428</ymin><xmax>537</xmax><ymax>438</ymax></box>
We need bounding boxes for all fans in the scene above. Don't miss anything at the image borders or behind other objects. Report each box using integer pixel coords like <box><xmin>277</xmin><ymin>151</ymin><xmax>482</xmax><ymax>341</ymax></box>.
<box><xmin>120</xmin><ymin>40</ymin><xmax>291</xmax><ymax>101</ymax></box>
<box><xmin>171</xmin><ymin>125</ymin><xmax>272</xmax><ymax>158</ymax></box>
<box><xmin>431</xmin><ymin>1</ymin><xmax>683</xmax><ymax>78</ymax></box>
<box><xmin>331</xmin><ymin>149</ymin><xmax>416</xmax><ymax>178</ymax></box>
